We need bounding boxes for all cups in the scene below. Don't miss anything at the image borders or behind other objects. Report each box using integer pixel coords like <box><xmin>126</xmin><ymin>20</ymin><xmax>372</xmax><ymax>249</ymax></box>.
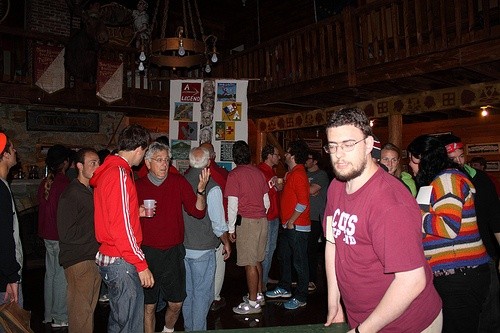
<box><xmin>143</xmin><ymin>200</ymin><xmax>155</xmax><ymax>217</ymax></box>
<box><xmin>308</xmin><ymin>178</ymin><xmax>314</xmax><ymax>187</ymax></box>
<box><xmin>276</xmin><ymin>177</ymin><xmax>283</xmax><ymax>191</ymax></box>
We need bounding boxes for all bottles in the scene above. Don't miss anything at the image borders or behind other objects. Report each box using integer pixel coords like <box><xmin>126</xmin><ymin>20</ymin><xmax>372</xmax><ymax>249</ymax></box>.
<box><xmin>8</xmin><ymin>160</ymin><xmax>48</xmax><ymax>180</ymax></box>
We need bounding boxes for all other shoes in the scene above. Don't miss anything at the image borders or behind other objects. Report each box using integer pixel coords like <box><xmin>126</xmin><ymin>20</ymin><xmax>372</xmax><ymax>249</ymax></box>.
<box><xmin>210</xmin><ymin>296</ymin><xmax>226</xmax><ymax>311</ymax></box>
<box><xmin>53</xmin><ymin>319</ymin><xmax>68</xmax><ymax>326</ymax></box>
<box><xmin>308</xmin><ymin>282</ymin><xmax>316</xmax><ymax>291</ymax></box>
<box><xmin>99</xmin><ymin>294</ymin><xmax>110</xmax><ymax>301</ymax></box>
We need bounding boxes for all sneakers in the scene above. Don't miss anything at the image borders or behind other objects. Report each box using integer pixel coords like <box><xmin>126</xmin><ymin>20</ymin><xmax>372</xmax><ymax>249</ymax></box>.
<box><xmin>233</xmin><ymin>299</ymin><xmax>261</xmax><ymax>315</ymax></box>
<box><xmin>284</xmin><ymin>298</ymin><xmax>306</xmax><ymax>309</ymax></box>
<box><xmin>243</xmin><ymin>293</ymin><xmax>267</xmax><ymax>306</ymax></box>
<box><xmin>266</xmin><ymin>288</ymin><xmax>292</xmax><ymax>298</ymax></box>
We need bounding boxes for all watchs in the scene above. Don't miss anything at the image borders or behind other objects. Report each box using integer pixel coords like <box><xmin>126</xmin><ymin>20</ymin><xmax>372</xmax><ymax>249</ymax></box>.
<box><xmin>197</xmin><ymin>188</ymin><xmax>206</xmax><ymax>195</ymax></box>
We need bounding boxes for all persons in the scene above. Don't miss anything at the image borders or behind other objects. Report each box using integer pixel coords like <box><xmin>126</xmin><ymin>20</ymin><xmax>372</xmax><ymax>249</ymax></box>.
<box><xmin>408</xmin><ymin>135</ymin><xmax>500</xmax><ymax>333</ymax></box>
<box><xmin>374</xmin><ymin>142</ymin><xmax>417</xmax><ymax>199</ymax></box>
<box><xmin>37</xmin><ymin>124</ymin><xmax>332</xmax><ymax>333</ymax></box>
<box><xmin>320</xmin><ymin>106</ymin><xmax>444</xmax><ymax>333</ymax></box>
<box><xmin>0</xmin><ymin>132</ymin><xmax>24</xmax><ymax>310</ymax></box>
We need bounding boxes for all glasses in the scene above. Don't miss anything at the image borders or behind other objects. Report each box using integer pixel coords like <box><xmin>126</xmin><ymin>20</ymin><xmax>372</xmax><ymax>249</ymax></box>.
<box><xmin>152</xmin><ymin>157</ymin><xmax>169</xmax><ymax>163</ymax></box>
<box><xmin>323</xmin><ymin>139</ymin><xmax>365</xmax><ymax>153</ymax></box>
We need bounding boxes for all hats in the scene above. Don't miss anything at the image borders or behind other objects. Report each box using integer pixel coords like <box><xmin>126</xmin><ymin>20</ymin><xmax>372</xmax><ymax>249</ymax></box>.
<box><xmin>0</xmin><ymin>133</ymin><xmax>7</xmax><ymax>154</ymax></box>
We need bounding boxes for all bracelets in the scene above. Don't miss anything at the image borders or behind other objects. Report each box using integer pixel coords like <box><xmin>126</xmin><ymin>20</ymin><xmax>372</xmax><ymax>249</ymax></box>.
<box><xmin>354</xmin><ymin>323</ymin><xmax>362</xmax><ymax>333</ymax></box>
